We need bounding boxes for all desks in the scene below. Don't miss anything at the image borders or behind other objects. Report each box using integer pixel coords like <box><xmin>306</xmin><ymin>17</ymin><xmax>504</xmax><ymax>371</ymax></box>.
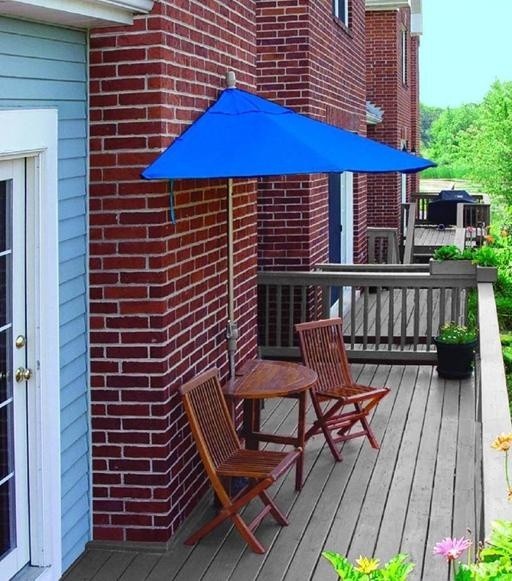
<box><xmin>219</xmin><ymin>358</ymin><xmax>319</xmax><ymax>495</ymax></box>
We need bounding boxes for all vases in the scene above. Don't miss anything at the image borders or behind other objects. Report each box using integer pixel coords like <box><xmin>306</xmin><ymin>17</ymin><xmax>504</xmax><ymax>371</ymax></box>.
<box><xmin>432</xmin><ymin>344</ymin><xmax>477</xmax><ymax>382</ymax></box>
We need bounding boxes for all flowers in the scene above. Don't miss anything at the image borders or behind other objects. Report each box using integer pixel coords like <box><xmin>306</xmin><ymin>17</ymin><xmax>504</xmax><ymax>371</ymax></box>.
<box><xmin>433</xmin><ymin>319</ymin><xmax>478</xmax><ymax>343</ymax></box>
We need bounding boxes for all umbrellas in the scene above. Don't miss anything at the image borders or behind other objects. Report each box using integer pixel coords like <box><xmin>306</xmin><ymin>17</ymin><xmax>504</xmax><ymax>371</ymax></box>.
<box><xmin>134</xmin><ymin>70</ymin><xmax>438</xmax><ymax>380</ymax></box>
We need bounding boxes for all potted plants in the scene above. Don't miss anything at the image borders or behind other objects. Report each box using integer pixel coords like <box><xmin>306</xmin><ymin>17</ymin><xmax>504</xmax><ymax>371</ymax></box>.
<box><xmin>474</xmin><ymin>247</ymin><xmax>499</xmax><ymax>284</ymax></box>
<box><xmin>427</xmin><ymin>245</ymin><xmax>476</xmax><ymax>275</ymax></box>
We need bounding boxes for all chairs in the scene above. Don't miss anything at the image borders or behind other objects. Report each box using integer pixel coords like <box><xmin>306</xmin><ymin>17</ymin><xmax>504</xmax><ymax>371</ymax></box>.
<box><xmin>178</xmin><ymin>367</ymin><xmax>303</xmax><ymax>555</ymax></box>
<box><xmin>294</xmin><ymin>317</ymin><xmax>391</xmax><ymax>466</ymax></box>
